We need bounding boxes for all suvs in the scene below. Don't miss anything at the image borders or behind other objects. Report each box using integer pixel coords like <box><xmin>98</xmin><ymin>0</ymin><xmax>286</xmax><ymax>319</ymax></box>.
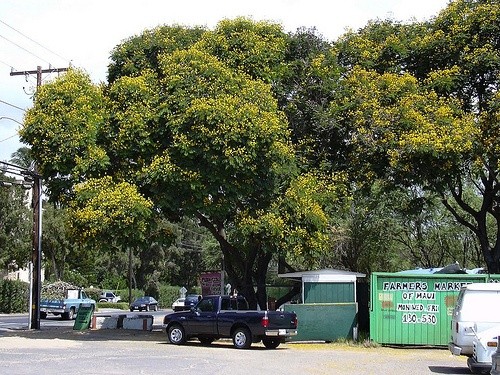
<box><xmin>184</xmin><ymin>295</ymin><xmax>202</xmax><ymax>309</ymax></box>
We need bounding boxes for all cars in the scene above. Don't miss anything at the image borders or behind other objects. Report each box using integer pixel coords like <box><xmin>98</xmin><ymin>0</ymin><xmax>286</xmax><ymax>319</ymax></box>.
<box><xmin>172</xmin><ymin>298</ymin><xmax>185</xmax><ymax>310</ymax></box>
<box><xmin>100</xmin><ymin>291</ymin><xmax>121</xmax><ymax>303</ymax></box>
<box><xmin>130</xmin><ymin>296</ymin><xmax>159</xmax><ymax>312</ymax></box>
<box><xmin>448</xmin><ymin>282</ymin><xmax>500</xmax><ymax>358</ymax></box>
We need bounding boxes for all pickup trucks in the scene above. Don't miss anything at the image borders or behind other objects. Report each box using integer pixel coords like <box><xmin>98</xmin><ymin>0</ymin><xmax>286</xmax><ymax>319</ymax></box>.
<box><xmin>39</xmin><ymin>290</ymin><xmax>97</xmax><ymax>320</ymax></box>
<box><xmin>162</xmin><ymin>294</ymin><xmax>298</xmax><ymax>350</ymax></box>
